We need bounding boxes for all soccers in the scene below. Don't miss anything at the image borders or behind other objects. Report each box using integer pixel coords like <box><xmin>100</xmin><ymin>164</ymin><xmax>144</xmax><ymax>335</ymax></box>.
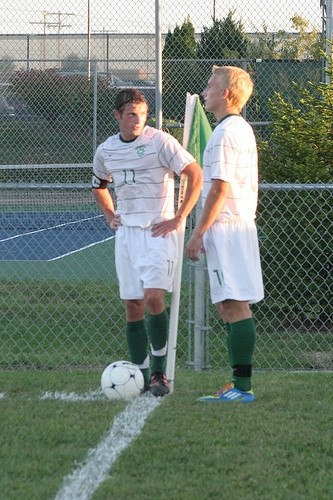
<box><xmin>100</xmin><ymin>361</ymin><xmax>146</xmax><ymax>404</ymax></box>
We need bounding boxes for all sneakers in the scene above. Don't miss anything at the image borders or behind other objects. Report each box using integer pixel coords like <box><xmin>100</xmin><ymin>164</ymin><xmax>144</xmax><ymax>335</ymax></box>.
<box><xmin>194</xmin><ymin>379</ymin><xmax>256</xmax><ymax>405</ymax></box>
<box><xmin>141</xmin><ymin>372</ymin><xmax>170</xmax><ymax>397</ymax></box>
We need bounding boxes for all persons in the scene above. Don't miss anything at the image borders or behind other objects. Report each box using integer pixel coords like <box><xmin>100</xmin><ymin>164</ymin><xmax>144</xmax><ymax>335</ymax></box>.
<box><xmin>184</xmin><ymin>65</ymin><xmax>265</xmax><ymax>403</ymax></box>
<box><xmin>92</xmin><ymin>89</ymin><xmax>203</xmax><ymax>396</ymax></box>
